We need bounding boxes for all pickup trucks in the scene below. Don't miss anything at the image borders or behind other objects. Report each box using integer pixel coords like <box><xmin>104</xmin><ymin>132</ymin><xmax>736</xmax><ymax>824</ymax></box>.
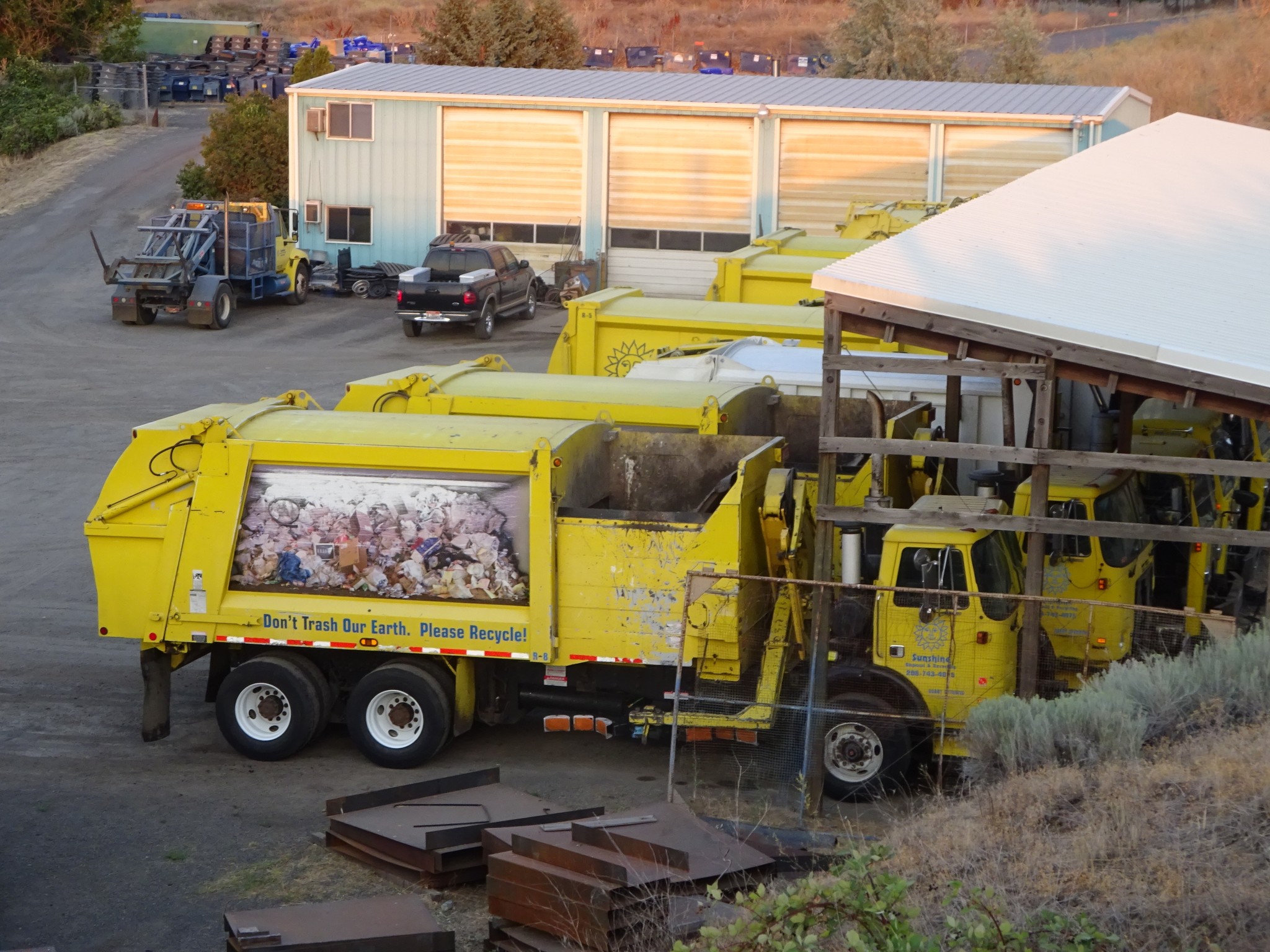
<box><xmin>393</xmin><ymin>241</ymin><xmax>538</xmax><ymax>341</ymax></box>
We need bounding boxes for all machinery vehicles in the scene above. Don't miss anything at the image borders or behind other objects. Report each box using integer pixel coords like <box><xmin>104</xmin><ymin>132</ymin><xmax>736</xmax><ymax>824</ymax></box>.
<box><xmin>90</xmin><ymin>190</ymin><xmax>315</xmax><ymax>330</ymax></box>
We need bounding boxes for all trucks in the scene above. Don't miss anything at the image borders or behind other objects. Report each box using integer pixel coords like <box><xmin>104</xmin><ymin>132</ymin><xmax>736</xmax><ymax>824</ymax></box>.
<box><xmin>549</xmin><ymin>286</ymin><xmax>1262</xmax><ymax>604</ymax></box>
<box><xmin>330</xmin><ymin>349</ymin><xmax>1161</xmax><ymax>702</ymax></box>
<box><xmin>705</xmin><ymin>224</ymin><xmax>1268</xmax><ymax>551</ymax></box>
<box><xmin>832</xmin><ymin>194</ymin><xmax>986</xmax><ymax>239</ymax></box>
<box><xmin>81</xmin><ymin>387</ymin><xmax>1024</xmax><ymax>809</ymax></box>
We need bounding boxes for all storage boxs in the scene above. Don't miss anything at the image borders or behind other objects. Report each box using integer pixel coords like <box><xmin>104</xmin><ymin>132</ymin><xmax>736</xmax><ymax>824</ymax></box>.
<box><xmin>387</xmin><ymin>494</ymin><xmax>416</xmax><ymax>520</ymax></box>
<box><xmin>581</xmin><ymin>45</ymin><xmax>849</xmax><ymax>77</ymax></box>
<box><xmin>79</xmin><ymin>11</ymin><xmax>417</xmax><ymax>109</ymax></box>
<box><xmin>339</xmin><ymin>510</ymin><xmax>374</xmax><ymax>573</ymax></box>
